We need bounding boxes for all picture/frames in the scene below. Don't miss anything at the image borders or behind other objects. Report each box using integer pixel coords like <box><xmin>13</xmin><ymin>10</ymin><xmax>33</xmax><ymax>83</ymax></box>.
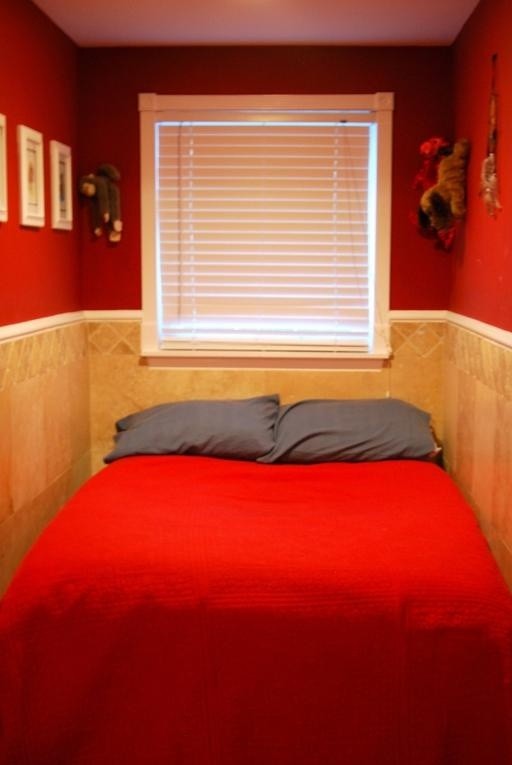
<box><xmin>0</xmin><ymin>112</ymin><xmax>75</xmax><ymax>232</ymax></box>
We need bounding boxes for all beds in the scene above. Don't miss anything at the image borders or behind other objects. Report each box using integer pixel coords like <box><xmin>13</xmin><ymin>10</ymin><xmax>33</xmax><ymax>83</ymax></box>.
<box><xmin>1</xmin><ymin>454</ymin><xmax>512</xmax><ymax>765</ymax></box>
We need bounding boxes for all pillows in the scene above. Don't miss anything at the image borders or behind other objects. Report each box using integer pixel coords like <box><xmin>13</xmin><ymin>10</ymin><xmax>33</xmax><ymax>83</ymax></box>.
<box><xmin>256</xmin><ymin>397</ymin><xmax>442</xmax><ymax>464</ymax></box>
<box><xmin>103</xmin><ymin>393</ymin><xmax>282</xmax><ymax>463</ymax></box>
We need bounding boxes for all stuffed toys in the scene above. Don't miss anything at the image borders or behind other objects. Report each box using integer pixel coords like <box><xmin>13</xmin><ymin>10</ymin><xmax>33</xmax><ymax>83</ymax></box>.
<box><xmin>414</xmin><ymin>138</ymin><xmax>454</xmax><ymax>251</ymax></box>
<box><xmin>419</xmin><ymin>137</ymin><xmax>469</xmax><ymax>230</ymax></box>
<box><xmin>79</xmin><ymin>164</ymin><xmax>123</xmax><ymax>243</ymax></box>
